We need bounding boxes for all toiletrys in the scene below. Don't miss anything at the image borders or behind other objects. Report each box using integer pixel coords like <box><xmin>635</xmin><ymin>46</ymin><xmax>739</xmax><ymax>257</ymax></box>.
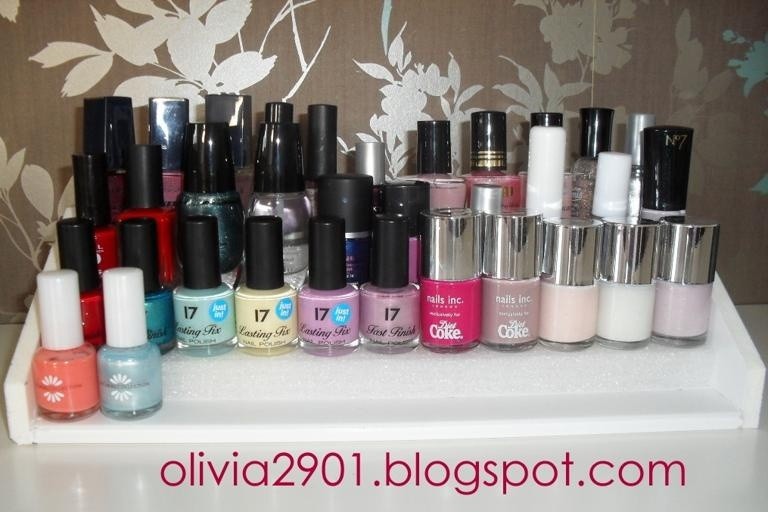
<box><xmin>30</xmin><ymin>92</ymin><xmax>720</xmax><ymax>421</ymax></box>
